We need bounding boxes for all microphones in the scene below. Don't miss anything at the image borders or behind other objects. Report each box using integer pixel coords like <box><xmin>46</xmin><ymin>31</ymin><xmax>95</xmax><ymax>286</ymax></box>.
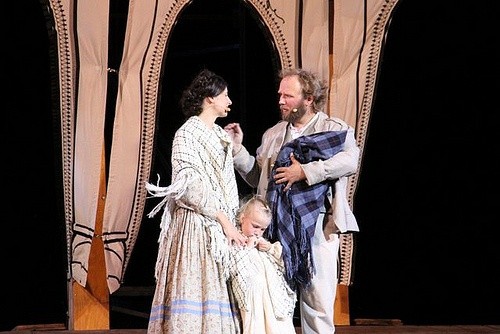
<box><xmin>210</xmin><ymin>104</ymin><xmax>230</xmax><ymax>111</ymax></box>
<box><xmin>293</xmin><ymin>109</ymin><xmax>298</xmax><ymax>113</ymax></box>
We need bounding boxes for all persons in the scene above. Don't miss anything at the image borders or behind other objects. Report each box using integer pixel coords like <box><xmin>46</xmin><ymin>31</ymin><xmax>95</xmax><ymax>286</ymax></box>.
<box><xmin>222</xmin><ymin>197</ymin><xmax>297</xmax><ymax>334</ymax></box>
<box><xmin>147</xmin><ymin>71</ymin><xmax>248</xmax><ymax>334</ymax></box>
<box><xmin>224</xmin><ymin>68</ymin><xmax>361</xmax><ymax>334</ymax></box>
